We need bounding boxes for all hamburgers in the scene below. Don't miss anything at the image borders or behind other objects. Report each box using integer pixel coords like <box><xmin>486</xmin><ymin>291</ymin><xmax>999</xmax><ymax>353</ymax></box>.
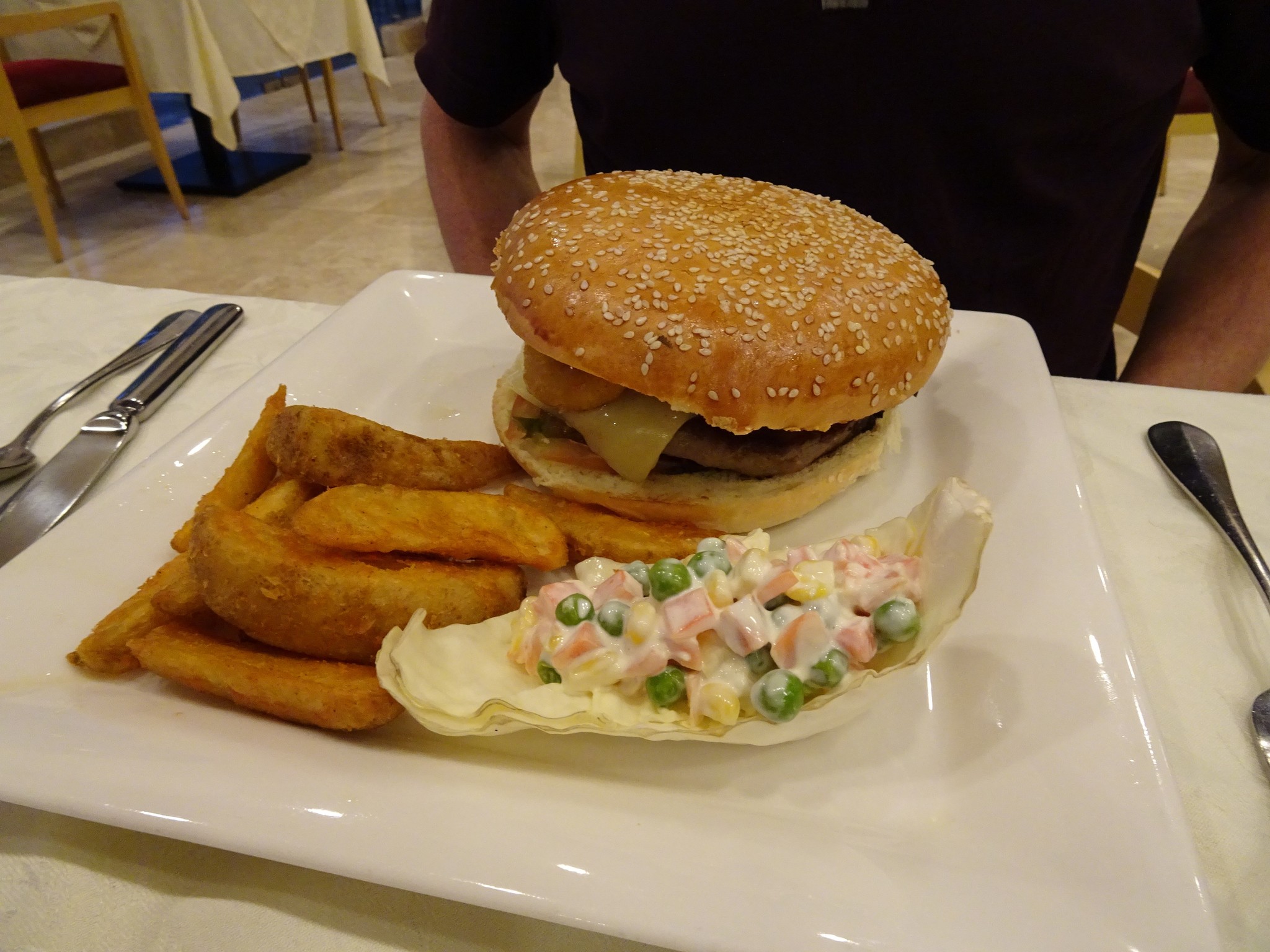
<box><xmin>491</xmin><ymin>168</ymin><xmax>952</xmax><ymax>532</ymax></box>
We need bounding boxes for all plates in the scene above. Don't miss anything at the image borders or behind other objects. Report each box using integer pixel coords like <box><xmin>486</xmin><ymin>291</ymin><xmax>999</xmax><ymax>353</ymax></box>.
<box><xmin>4</xmin><ymin>266</ymin><xmax>1217</xmax><ymax>952</ymax></box>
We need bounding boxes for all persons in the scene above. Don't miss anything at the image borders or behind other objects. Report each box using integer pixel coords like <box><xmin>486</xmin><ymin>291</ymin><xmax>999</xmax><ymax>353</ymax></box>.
<box><xmin>411</xmin><ymin>2</ymin><xmax>1270</xmax><ymax>395</ymax></box>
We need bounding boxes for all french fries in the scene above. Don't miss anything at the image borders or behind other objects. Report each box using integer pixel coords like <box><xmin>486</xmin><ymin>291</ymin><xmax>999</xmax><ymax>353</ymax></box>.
<box><xmin>66</xmin><ymin>384</ymin><xmax>710</xmax><ymax>732</ymax></box>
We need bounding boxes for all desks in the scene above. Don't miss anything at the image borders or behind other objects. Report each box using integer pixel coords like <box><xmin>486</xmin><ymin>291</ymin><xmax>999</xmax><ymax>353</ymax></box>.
<box><xmin>1</xmin><ymin>0</ymin><xmax>390</xmax><ymax>199</ymax></box>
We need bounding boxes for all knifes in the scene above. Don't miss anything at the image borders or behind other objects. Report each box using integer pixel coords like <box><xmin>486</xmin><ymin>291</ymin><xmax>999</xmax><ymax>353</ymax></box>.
<box><xmin>2</xmin><ymin>295</ymin><xmax>248</xmax><ymax>562</ymax></box>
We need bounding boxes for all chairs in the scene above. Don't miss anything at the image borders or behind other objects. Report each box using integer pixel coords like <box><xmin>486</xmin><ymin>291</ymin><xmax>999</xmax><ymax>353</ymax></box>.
<box><xmin>1</xmin><ymin>0</ymin><xmax>190</xmax><ymax>265</ymax></box>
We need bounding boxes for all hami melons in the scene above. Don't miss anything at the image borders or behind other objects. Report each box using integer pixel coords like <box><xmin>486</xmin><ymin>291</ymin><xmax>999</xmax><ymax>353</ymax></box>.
<box><xmin>512</xmin><ymin>525</ymin><xmax>925</xmax><ymax>727</ymax></box>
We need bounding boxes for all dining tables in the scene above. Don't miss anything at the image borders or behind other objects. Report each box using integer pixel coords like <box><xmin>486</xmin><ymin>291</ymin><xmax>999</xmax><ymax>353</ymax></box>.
<box><xmin>0</xmin><ymin>273</ymin><xmax>1270</xmax><ymax>952</ymax></box>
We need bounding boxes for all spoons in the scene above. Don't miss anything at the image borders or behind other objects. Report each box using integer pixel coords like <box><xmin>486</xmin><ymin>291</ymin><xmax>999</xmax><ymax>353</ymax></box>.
<box><xmin>2</xmin><ymin>307</ymin><xmax>207</xmax><ymax>484</ymax></box>
<box><xmin>1133</xmin><ymin>414</ymin><xmax>1270</xmax><ymax>775</ymax></box>
